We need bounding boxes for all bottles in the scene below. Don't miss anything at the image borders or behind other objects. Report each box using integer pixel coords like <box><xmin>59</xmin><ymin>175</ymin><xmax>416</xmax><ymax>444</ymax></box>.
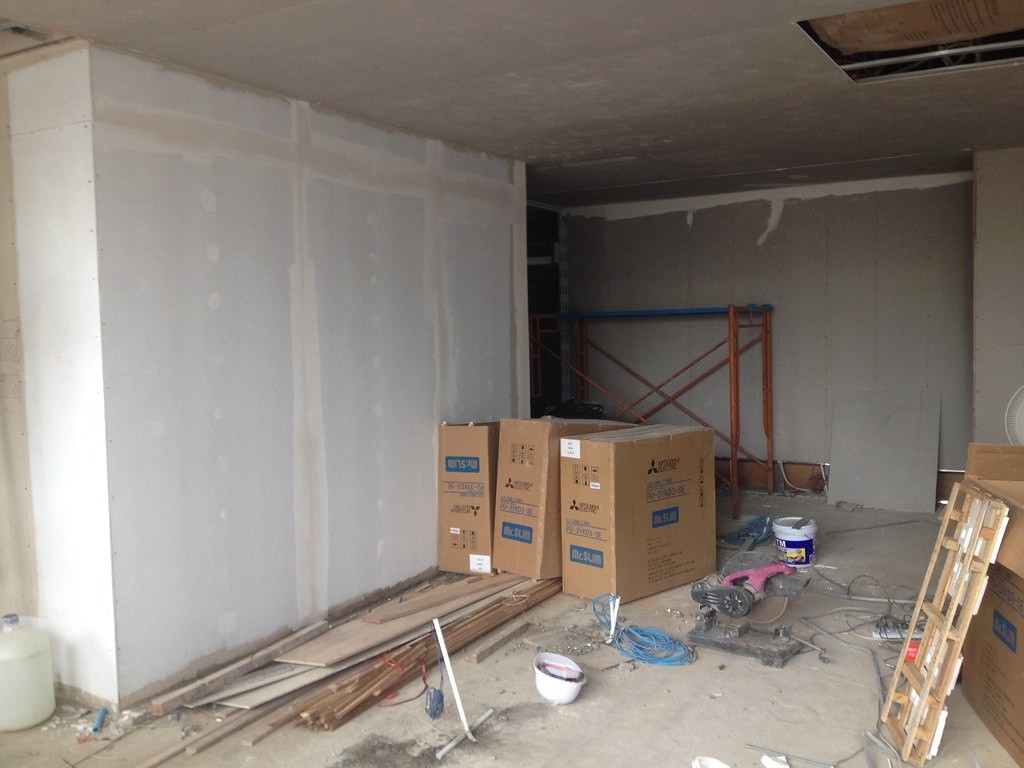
<box><xmin>1</xmin><ymin>613</ymin><xmax>56</xmax><ymax>734</ymax></box>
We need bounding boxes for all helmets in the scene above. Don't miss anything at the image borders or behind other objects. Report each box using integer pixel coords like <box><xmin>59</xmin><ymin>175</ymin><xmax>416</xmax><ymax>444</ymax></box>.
<box><xmin>531</xmin><ymin>655</ymin><xmax>590</xmax><ymax>706</ymax></box>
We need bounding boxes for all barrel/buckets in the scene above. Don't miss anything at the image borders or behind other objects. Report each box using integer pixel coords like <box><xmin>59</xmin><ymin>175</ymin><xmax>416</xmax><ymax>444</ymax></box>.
<box><xmin>773</xmin><ymin>517</ymin><xmax>817</xmax><ymax>567</ymax></box>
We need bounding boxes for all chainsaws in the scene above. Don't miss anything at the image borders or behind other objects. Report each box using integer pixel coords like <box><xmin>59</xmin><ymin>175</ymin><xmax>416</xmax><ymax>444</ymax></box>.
<box><xmin>686</xmin><ymin>550</ymin><xmax>810</xmax><ymax>667</ymax></box>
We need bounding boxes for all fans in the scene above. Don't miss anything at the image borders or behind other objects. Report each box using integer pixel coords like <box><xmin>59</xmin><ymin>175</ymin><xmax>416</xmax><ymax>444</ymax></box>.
<box><xmin>1004</xmin><ymin>384</ymin><xmax>1023</xmax><ymax>445</ymax></box>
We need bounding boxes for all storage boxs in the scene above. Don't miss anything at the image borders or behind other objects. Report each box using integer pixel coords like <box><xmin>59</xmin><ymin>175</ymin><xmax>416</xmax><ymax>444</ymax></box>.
<box><xmin>560</xmin><ymin>424</ymin><xmax>717</xmax><ymax>606</ymax></box>
<box><xmin>494</xmin><ymin>416</ymin><xmax>643</xmax><ymax>580</ymax></box>
<box><xmin>961</xmin><ymin>442</ymin><xmax>1024</xmax><ymax>768</ymax></box>
<box><xmin>439</xmin><ymin>422</ymin><xmax>500</xmax><ymax>576</ymax></box>
